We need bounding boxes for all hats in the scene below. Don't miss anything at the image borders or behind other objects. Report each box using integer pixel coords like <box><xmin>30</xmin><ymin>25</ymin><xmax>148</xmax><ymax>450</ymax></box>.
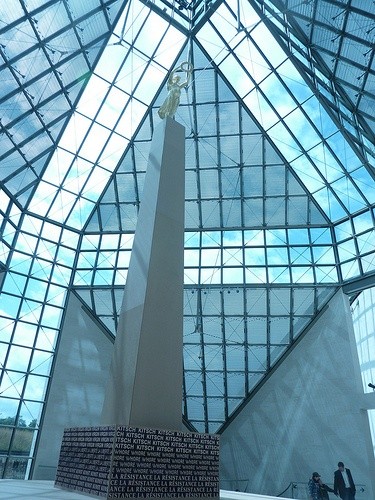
<box><xmin>312</xmin><ymin>471</ymin><xmax>320</xmax><ymax>478</ymax></box>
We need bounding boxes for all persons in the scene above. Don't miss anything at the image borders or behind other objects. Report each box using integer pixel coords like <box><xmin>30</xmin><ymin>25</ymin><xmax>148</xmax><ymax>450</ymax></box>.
<box><xmin>308</xmin><ymin>472</ymin><xmax>339</xmax><ymax>500</ymax></box>
<box><xmin>333</xmin><ymin>461</ymin><xmax>357</xmax><ymax>500</ymax></box>
